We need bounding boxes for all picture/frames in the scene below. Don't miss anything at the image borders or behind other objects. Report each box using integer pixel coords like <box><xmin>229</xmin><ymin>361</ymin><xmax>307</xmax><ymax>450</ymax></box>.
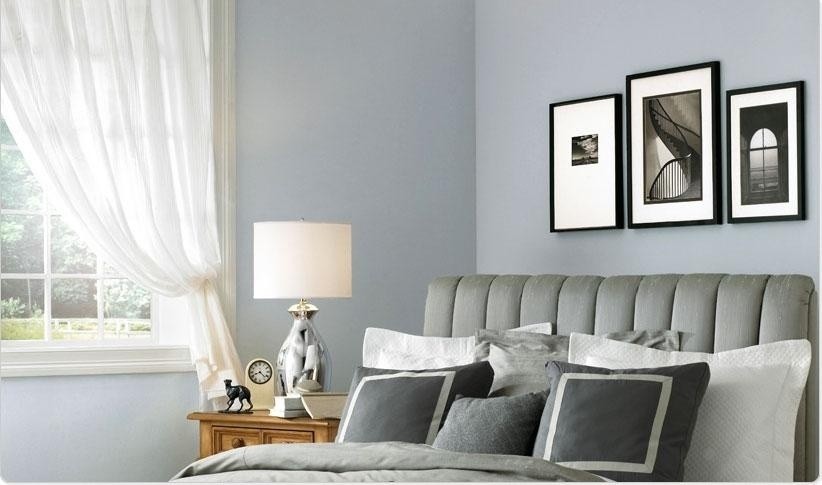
<box><xmin>726</xmin><ymin>80</ymin><xmax>807</xmax><ymax>223</ymax></box>
<box><xmin>625</xmin><ymin>59</ymin><xmax>724</xmax><ymax>228</ymax></box>
<box><xmin>548</xmin><ymin>93</ymin><xmax>624</xmax><ymax>233</ymax></box>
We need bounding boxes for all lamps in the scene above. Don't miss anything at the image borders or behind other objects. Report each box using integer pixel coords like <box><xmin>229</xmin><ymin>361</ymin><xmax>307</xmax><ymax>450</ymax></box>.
<box><xmin>253</xmin><ymin>221</ymin><xmax>353</xmax><ymax>416</ymax></box>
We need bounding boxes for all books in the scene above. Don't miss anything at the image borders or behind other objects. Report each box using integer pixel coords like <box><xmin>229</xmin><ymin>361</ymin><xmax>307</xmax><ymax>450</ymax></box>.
<box><xmin>268</xmin><ymin>391</ymin><xmax>349</xmax><ymax>422</ymax></box>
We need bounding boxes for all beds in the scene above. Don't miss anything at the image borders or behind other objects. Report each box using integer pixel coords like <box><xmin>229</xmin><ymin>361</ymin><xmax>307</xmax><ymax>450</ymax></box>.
<box><xmin>169</xmin><ymin>275</ymin><xmax>818</xmax><ymax>480</ymax></box>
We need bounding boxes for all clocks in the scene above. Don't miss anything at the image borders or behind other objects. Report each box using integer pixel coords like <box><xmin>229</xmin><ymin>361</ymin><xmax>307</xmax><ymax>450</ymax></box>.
<box><xmin>241</xmin><ymin>358</ymin><xmax>276</xmax><ymax>411</ymax></box>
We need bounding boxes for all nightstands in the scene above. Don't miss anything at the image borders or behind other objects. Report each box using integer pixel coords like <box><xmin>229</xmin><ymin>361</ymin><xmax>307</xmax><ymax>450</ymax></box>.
<box><xmin>187</xmin><ymin>412</ymin><xmax>342</xmax><ymax>460</ymax></box>
